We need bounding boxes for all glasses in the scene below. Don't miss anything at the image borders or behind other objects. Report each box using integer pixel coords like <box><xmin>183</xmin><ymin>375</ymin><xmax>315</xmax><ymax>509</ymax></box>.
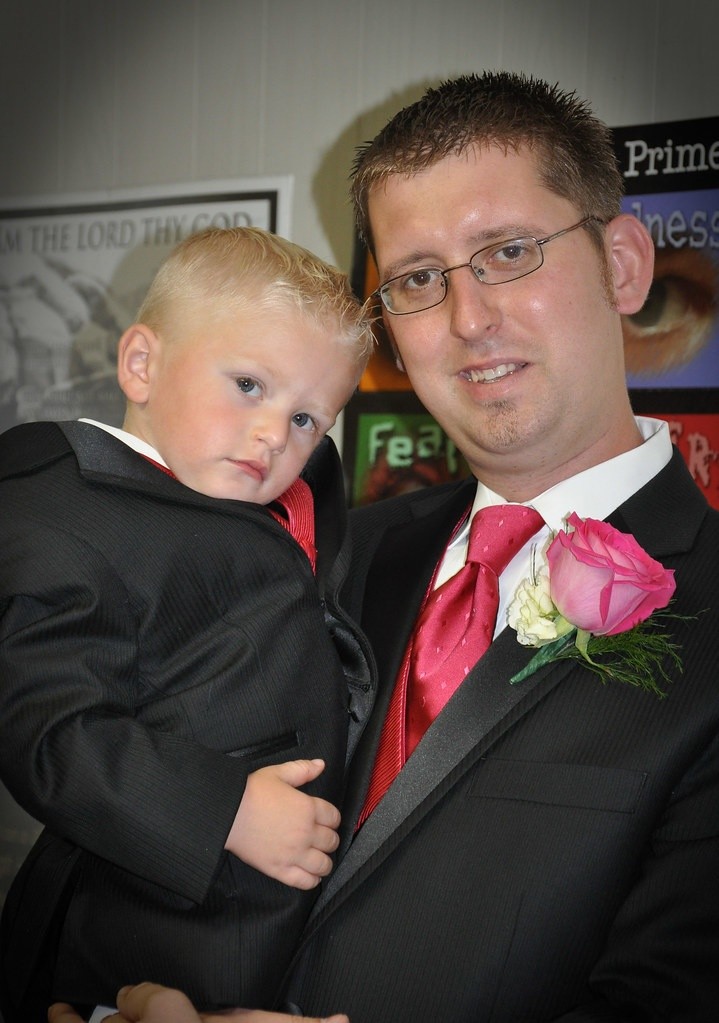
<box><xmin>370</xmin><ymin>216</ymin><xmax>611</xmax><ymax>316</ymax></box>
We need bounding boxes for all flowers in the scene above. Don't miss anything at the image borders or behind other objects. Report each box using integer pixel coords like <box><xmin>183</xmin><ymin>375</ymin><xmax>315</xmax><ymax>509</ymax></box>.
<box><xmin>505</xmin><ymin>512</ymin><xmax>711</xmax><ymax>702</ymax></box>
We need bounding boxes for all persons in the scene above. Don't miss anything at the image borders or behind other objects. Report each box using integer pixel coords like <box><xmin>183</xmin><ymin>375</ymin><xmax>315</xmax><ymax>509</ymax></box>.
<box><xmin>0</xmin><ymin>228</ymin><xmax>372</xmax><ymax>1022</ymax></box>
<box><xmin>48</xmin><ymin>69</ymin><xmax>718</xmax><ymax>1023</ymax></box>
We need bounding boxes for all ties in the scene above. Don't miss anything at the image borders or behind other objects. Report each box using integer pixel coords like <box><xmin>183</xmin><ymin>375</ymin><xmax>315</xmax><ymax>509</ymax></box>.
<box><xmin>403</xmin><ymin>504</ymin><xmax>547</xmax><ymax>767</ymax></box>
